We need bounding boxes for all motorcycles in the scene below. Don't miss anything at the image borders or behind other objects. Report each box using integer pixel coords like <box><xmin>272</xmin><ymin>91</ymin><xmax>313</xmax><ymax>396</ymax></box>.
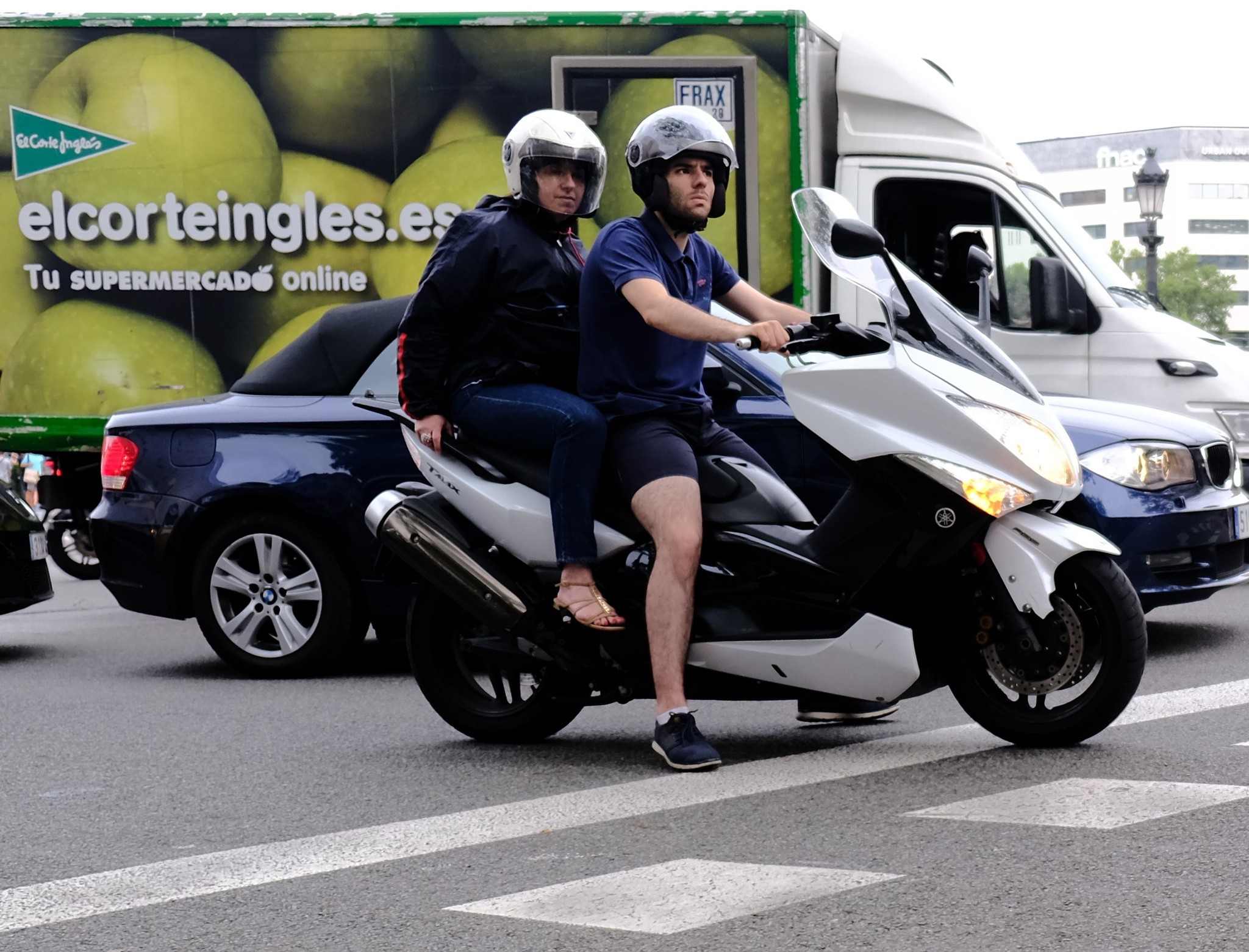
<box><xmin>356</xmin><ymin>186</ymin><xmax>1152</xmax><ymax>751</ymax></box>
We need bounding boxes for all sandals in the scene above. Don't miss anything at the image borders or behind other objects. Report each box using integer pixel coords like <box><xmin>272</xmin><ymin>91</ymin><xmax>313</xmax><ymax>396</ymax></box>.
<box><xmin>553</xmin><ymin>580</ymin><xmax>627</xmax><ymax>631</ymax></box>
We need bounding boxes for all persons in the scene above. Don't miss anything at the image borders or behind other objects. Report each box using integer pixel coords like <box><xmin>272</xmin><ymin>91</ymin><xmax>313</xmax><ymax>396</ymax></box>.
<box><xmin>398</xmin><ymin>110</ymin><xmax>624</xmax><ymax>631</ymax></box>
<box><xmin>0</xmin><ymin>451</ymin><xmax>61</xmax><ymax>511</ymax></box>
<box><xmin>936</xmin><ymin>231</ymin><xmax>1030</xmax><ymax>328</ymax></box>
<box><xmin>568</xmin><ymin>105</ymin><xmax>815</xmax><ymax>770</ymax></box>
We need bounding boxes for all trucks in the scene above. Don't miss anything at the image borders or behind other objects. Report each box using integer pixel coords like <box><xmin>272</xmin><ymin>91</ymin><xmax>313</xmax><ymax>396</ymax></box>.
<box><xmin>0</xmin><ymin>11</ymin><xmax>1249</xmax><ymax>498</ymax></box>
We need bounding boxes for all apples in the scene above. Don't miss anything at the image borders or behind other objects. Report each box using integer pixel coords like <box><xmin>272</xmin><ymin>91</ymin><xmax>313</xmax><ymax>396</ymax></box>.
<box><xmin>0</xmin><ymin>23</ymin><xmax>794</xmax><ymax>414</ymax></box>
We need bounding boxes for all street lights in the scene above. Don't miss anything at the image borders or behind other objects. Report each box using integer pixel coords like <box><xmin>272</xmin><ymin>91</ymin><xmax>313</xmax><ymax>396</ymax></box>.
<box><xmin>1133</xmin><ymin>145</ymin><xmax>1171</xmax><ymax>306</ymax></box>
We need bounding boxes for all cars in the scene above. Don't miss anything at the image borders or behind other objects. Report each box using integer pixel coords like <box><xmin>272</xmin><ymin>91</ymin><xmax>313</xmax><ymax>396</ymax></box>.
<box><xmin>79</xmin><ymin>292</ymin><xmax>1249</xmax><ymax>675</ymax></box>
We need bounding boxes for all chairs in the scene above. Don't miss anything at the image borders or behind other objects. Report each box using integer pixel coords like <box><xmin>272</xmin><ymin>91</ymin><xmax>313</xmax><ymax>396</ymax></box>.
<box><xmin>903</xmin><ymin>208</ymin><xmax>945</xmax><ymax>291</ymax></box>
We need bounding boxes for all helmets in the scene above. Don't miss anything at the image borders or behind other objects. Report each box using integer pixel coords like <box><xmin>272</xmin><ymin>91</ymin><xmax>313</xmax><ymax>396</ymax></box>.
<box><xmin>501</xmin><ymin>109</ymin><xmax>605</xmax><ymax>199</ymax></box>
<box><xmin>625</xmin><ymin>106</ymin><xmax>732</xmax><ymax>220</ymax></box>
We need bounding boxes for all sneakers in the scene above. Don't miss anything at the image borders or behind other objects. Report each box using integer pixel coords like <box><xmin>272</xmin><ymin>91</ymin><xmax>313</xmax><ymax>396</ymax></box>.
<box><xmin>796</xmin><ymin>698</ymin><xmax>901</xmax><ymax>722</ymax></box>
<box><xmin>651</xmin><ymin>709</ymin><xmax>723</xmax><ymax>769</ymax></box>
